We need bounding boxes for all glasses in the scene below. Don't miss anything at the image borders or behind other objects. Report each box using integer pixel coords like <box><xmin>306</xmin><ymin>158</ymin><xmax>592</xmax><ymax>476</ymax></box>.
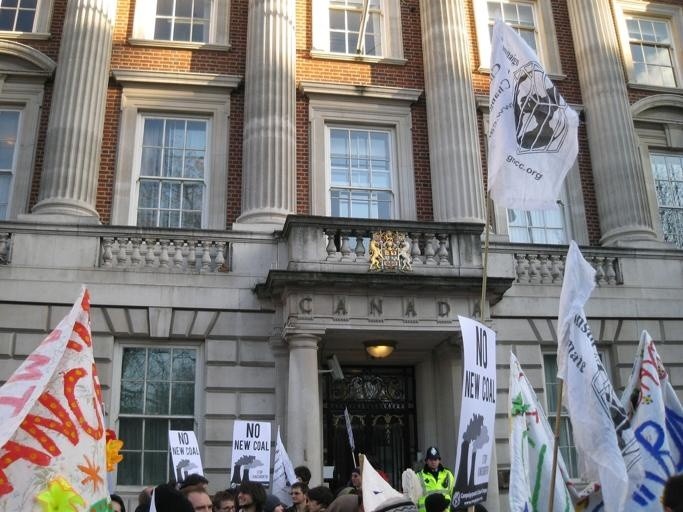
<box><xmin>290</xmin><ymin>492</ymin><xmax>299</xmax><ymax>495</ymax></box>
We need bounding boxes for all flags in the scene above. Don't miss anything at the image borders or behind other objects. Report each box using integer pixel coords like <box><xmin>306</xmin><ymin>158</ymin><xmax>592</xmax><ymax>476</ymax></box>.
<box><xmin>486</xmin><ymin>11</ymin><xmax>580</xmax><ymax>210</ymax></box>
<box><xmin>0</xmin><ymin>285</ymin><xmax>113</xmax><ymax>512</ymax></box>
<box><xmin>271</xmin><ymin>431</ymin><xmax>300</xmax><ymax>510</ymax></box>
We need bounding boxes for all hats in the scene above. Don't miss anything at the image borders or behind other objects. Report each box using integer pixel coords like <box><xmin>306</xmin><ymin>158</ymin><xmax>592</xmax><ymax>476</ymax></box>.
<box><xmin>148</xmin><ymin>484</ymin><xmax>195</xmax><ymax>512</ymax></box>
<box><xmin>425</xmin><ymin>493</ymin><xmax>450</xmax><ymax>512</ymax></box>
<box><xmin>425</xmin><ymin>447</ymin><xmax>441</xmax><ymax>462</ymax></box>
<box><xmin>373</xmin><ymin>498</ymin><xmax>419</xmax><ymax>512</ymax></box>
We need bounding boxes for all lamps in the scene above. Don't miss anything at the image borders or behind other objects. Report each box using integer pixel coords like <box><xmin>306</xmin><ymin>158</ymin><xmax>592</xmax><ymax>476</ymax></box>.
<box><xmin>361</xmin><ymin>338</ymin><xmax>398</xmax><ymax>359</ymax></box>
